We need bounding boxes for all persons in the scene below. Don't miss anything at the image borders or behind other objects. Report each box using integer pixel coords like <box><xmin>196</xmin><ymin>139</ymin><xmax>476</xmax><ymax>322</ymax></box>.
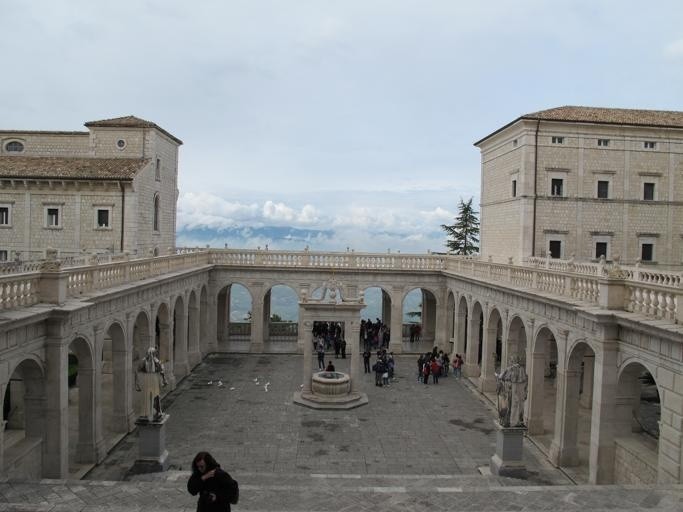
<box><xmin>360</xmin><ymin>316</ymin><xmax>397</xmax><ymax>388</ymax></box>
<box><xmin>312</xmin><ymin>320</ymin><xmax>347</xmax><ymax>373</ymax></box>
<box><xmin>409</xmin><ymin>323</ymin><xmax>416</xmax><ymax>342</ymax></box>
<box><xmin>415</xmin><ymin>325</ymin><xmax>421</xmax><ymax>341</ymax></box>
<box><xmin>187</xmin><ymin>452</ymin><xmax>239</xmax><ymax>512</ymax></box>
<box><xmin>416</xmin><ymin>345</ymin><xmax>462</xmax><ymax>385</ymax></box>
<box><xmin>495</xmin><ymin>355</ymin><xmax>527</xmax><ymax>428</ymax></box>
<box><xmin>134</xmin><ymin>347</ymin><xmax>168</xmax><ymax>419</ymax></box>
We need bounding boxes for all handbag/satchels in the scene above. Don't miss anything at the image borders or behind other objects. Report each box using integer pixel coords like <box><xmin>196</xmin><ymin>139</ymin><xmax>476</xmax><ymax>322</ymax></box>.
<box><xmin>229</xmin><ymin>480</ymin><xmax>239</xmax><ymax>504</ymax></box>
